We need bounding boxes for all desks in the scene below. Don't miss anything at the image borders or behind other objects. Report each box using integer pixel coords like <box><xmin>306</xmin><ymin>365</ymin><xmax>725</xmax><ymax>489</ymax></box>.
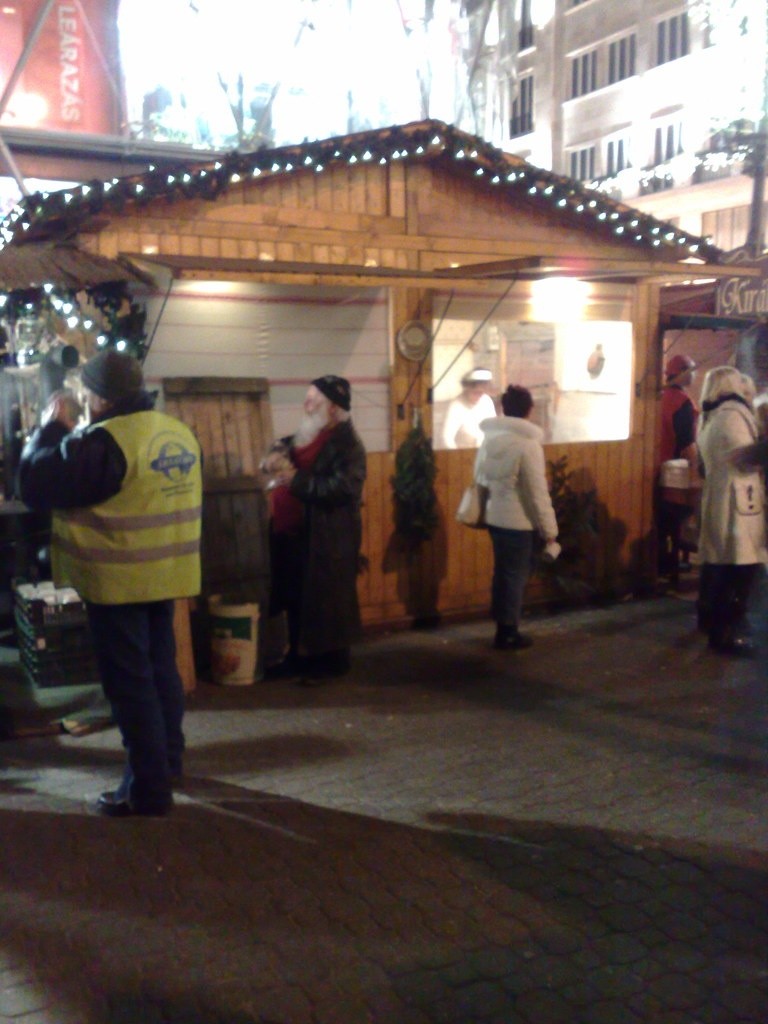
<box><xmin>661</xmin><ymin>481</ymin><xmax>705</xmax><ymax>581</ymax></box>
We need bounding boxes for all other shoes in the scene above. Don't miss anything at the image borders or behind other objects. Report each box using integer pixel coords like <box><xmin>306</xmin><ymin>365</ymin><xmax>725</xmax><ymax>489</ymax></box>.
<box><xmin>493</xmin><ymin>631</ymin><xmax>534</xmax><ymax>648</ymax></box>
<box><xmin>710</xmin><ymin>627</ymin><xmax>757</xmax><ymax>656</ymax></box>
<box><xmin>737</xmin><ymin>612</ymin><xmax>758</xmax><ymax>637</ymax></box>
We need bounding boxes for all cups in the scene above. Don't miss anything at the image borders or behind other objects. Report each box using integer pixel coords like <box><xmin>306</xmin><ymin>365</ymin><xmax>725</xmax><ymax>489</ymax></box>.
<box><xmin>540</xmin><ymin>540</ymin><xmax>563</xmax><ymax>562</ymax></box>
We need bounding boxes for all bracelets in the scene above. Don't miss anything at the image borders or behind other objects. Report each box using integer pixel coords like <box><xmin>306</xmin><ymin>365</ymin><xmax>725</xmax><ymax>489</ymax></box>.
<box><xmin>259</xmin><ymin>459</ymin><xmax>268</xmax><ymax>473</ymax></box>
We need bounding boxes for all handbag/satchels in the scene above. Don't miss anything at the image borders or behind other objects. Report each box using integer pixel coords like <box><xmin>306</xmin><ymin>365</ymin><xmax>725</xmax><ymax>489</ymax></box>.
<box><xmin>659</xmin><ymin>458</ymin><xmax>705</xmax><ymax>488</ymax></box>
<box><xmin>454</xmin><ymin>461</ymin><xmax>487</xmax><ymax>529</ymax></box>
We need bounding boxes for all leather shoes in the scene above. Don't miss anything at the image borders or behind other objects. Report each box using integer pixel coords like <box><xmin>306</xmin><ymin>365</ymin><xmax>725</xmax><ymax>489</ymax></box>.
<box><xmin>97</xmin><ymin>791</ymin><xmax>174</xmax><ymax>814</ymax></box>
<box><xmin>172</xmin><ymin>760</ymin><xmax>185</xmax><ymax>780</ymax></box>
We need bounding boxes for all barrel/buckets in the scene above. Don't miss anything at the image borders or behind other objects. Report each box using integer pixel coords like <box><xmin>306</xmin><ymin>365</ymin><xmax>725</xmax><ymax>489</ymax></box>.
<box><xmin>208</xmin><ymin>593</ymin><xmax>259</xmax><ymax>687</ymax></box>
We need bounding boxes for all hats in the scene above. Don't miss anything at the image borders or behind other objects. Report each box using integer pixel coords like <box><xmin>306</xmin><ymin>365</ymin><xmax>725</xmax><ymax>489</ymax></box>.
<box><xmin>667</xmin><ymin>354</ymin><xmax>689</xmax><ymax>374</ymax></box>
<box><xmin>313</xmin><ymin>375</ymin><xmax>350</xmax><ymax>412</ymax></box>
<box><xmin>80</xmin><ymin>348</ymin><xmax>143</xmax><ymax>401</ymax></box>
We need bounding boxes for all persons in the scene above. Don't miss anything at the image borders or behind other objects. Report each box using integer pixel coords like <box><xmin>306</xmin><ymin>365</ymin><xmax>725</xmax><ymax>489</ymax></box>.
<box><xmin>652</xmin><ymin>353</ymin><xmax>705</xmax><ymax>572</ymax></box>
<box><xmin>472</xmin><ymin>383</ymin><xmax>560</xmax><ymax>647</ymax></box>
<box><xmin>695</xmin><ymin>363</ymin><xmax>768</xmax><ymax>659</ymax></box>
<box><xmin>258</xmin><ymin>374</ymin><xmax>369</xmax><ymax>684</ymax></box>
<box><xmin>14</xmin><ymin>350</ymin><xmax>205</xmax><ymax>817</ymax></box>
<box><xmin>442</xmin><ymin>369</ymin><xmax>500</xmax><ymax>450</ymax></box>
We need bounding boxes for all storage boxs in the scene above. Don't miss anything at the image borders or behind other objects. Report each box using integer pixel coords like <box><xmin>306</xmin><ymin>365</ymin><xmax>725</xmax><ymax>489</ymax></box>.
<box><xmin>12</xmin><ymin>580</ymin><xmax>99</xmax><ymax>686</ymax></box>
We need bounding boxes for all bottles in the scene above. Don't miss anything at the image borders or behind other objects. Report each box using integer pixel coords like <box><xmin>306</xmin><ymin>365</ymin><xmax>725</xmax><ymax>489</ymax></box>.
<box><xmin>39</xmin><ymin>395</ymin><xmax>87</xmax><ymax>429</ymax></box>
